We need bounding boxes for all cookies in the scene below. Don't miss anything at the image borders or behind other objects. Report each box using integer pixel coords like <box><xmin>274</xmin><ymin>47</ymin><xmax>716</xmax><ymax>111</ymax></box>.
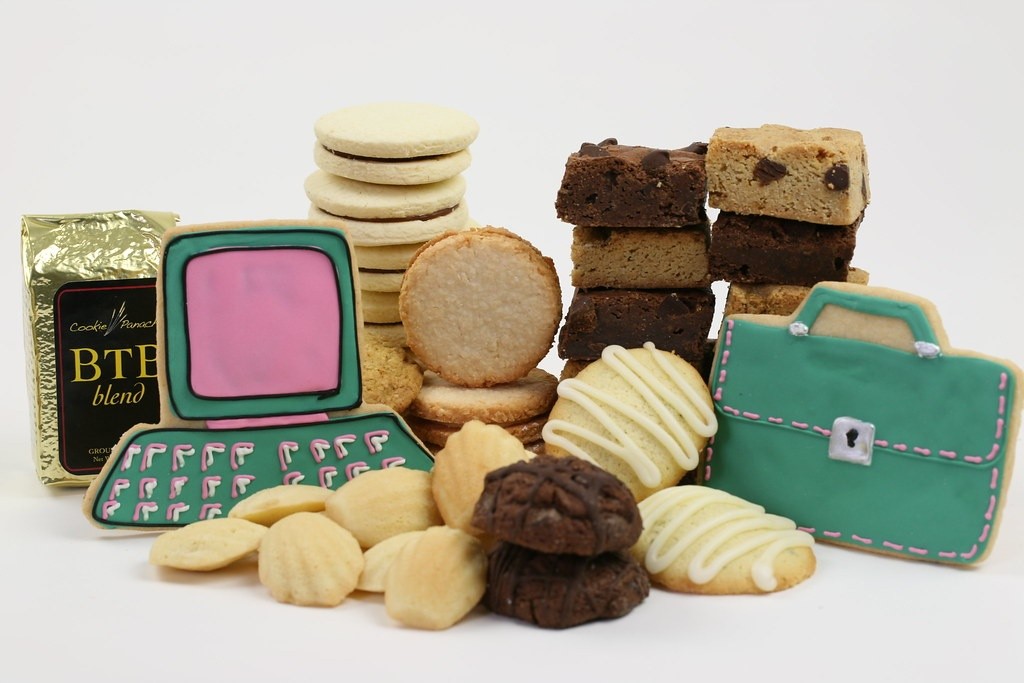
<box><xmin>82</xmin><ymin>100</ymin><xmax>816</xmax><ymax>630</ymax></box>
<box><xmin>692</xmin><ymin>283</ymin><xmax>1024</xmax><ymax>563</ymax></box>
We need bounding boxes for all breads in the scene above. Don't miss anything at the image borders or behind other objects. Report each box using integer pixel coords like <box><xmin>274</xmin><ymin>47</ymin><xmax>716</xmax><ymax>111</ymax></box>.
<box><xmin>556</xmin><ymin>124</ymin><xmax>870</xmax><ymax>386</ymax></box>
